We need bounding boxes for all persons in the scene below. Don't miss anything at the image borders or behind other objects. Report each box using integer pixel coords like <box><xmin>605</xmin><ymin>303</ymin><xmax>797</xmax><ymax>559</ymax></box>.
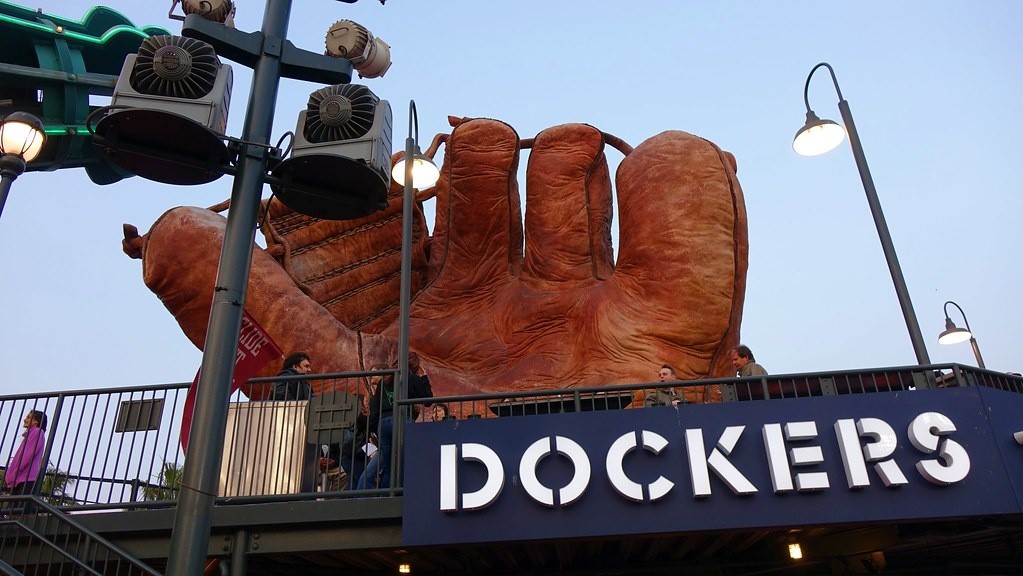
<box><xmin>315</xmin><ymin>350</ymin><xmax>449</xmax><ymax>501</ymax></box>
<box><xmin>4</xmin><ymin>410</ymin><xmax>47</xmax><ymax>514</ymax></box>
<box><xmin>730</xmin><ymin>344</ymin><xmax>769</xmax><ymax>377</ymax></box>
<box><xmin>267</xmin><ymin>352</ymin><xmax>314</xmax><ymax>401</ymax></box>
<box><xmin>644</xmin><ymin>364</ymin><xmax>689</xmax><ymax>407</ymax></box>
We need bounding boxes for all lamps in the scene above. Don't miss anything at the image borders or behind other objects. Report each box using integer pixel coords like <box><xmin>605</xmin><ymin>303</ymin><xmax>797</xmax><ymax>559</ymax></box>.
<box><xmin>397</xmin><ymin>554</ymin><xmax>411</xmax><ymax>574</ymax></box>
<box><xmin>785</xmin><ymin>535</ymin><xmax>803</xmax><ymax>559</ymax></box>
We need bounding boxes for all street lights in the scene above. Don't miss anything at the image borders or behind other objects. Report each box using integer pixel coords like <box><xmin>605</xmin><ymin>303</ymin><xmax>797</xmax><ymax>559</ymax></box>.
<box><xmin>938</xmin><ymin>300</ymin><xmax>986</xmax><ymax>370</ymax></box>
<box><xmin>387</xmin><ymin>101</ymin><xmax>442</xmax><ymax>489</ymax></box>
<box><xmin>792</xmin><ymin>60</ymin><xmax>938</xmax><ymax>388</ymax></box>
<box><xmin>0</xmin><ymin>111</ymin><xmax>48</xmax><ymax>219</ymax></box>
